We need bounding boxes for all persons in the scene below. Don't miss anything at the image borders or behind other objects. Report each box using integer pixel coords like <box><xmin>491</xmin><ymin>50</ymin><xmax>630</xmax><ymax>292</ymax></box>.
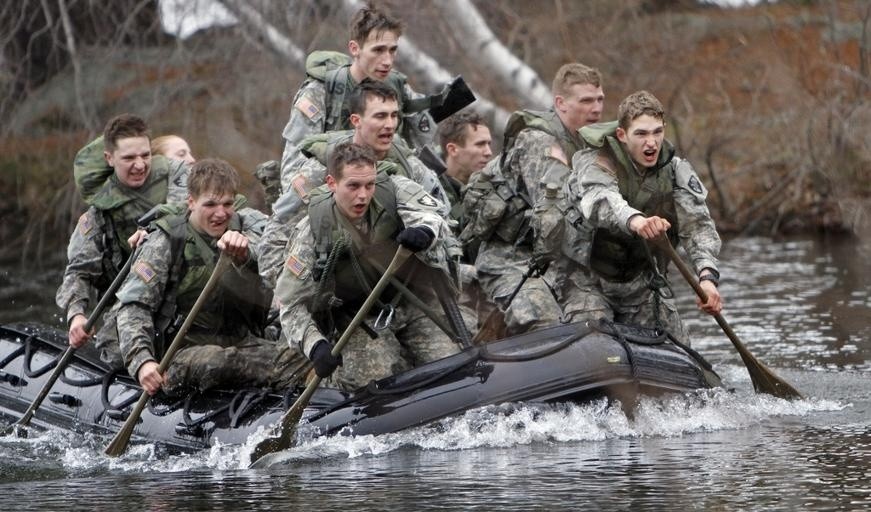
<box><xmin>278</xmin><ymin>0</ymin><xmax>439</xmax><ymax>156</ymax></box>
<box><xmin>562</xmin><ymin>90</ymin><xmax>723</xmax><ymax>321</ymax></box>
<box><xmin>257</xmin><ymin>77</ymin><xmax>452</xmax><ymax>292</ymax></box>
<box><xmin>149</xmin><ymin>133</ymin><xmax>271</xmax><ymax>235</ymax></box>
<box><xmin>459</xmin><ymin>63</ymin><xmax>605</xmax><ymax>343</ymax></box>
<box><xmin>55</xmin><ymin>115</ymin><xmax>195</xmax><ymax>348</ymax></box>
<box><xmin>275</xmin><ymin>141</ymin><xmax>464</xmax><ymax>395</ymax></box>
<box><xmin>432</xmin><ymin>106</ymin><xmax>495</xmax><ymax>203</ymax></box>
<box><xmin>110</xmin><ymin>158</ymin><xmax>313</xmax><ymax>398</ymax></box>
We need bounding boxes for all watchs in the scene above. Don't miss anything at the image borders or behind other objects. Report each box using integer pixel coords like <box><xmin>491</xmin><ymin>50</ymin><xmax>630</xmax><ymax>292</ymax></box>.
<box><xmin>699</xmin><ymin>272</ymin><xmax>719</xmax><ymax>287</ymax></box>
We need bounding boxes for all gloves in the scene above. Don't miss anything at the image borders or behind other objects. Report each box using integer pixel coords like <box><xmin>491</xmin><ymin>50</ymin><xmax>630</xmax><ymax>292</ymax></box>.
<box><xmin>396</xmin><ymin>226</ymin><xmax>435</xmax><ymax>252</ymax></box>
<box><xmin>309</xmin><ymin>339</ymin><xmax>343</xmax><ymax>378</ymax></box>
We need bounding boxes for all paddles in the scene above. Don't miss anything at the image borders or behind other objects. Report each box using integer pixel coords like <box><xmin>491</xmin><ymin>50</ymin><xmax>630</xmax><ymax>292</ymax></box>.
<box><xmin>0</xmin><ymin>249</ymin><xmax>134</xmax><ymax>437</ymax></box>
<box><xmin>649</xmin><ymin>223</ymin><xmax>802</xmax><ymax>400</ymax></box>
<box><xmin>250</xmin><ymin>245</ymin><xmax>413</xmax><ymax>467</ymax></box>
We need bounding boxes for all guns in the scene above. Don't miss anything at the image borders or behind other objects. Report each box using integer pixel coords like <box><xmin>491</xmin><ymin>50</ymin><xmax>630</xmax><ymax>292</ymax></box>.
<box><xmin>427</xmin><ymin>75</ymin><xmax>477</xmax><ymax>125</ymax></box>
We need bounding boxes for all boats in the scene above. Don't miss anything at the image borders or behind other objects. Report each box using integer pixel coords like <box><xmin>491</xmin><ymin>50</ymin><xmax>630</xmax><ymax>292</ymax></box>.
<box><xmin>0</xmin><ymin>320</ymin><xmax>729</xmax><ymax>462</ymax></box>
